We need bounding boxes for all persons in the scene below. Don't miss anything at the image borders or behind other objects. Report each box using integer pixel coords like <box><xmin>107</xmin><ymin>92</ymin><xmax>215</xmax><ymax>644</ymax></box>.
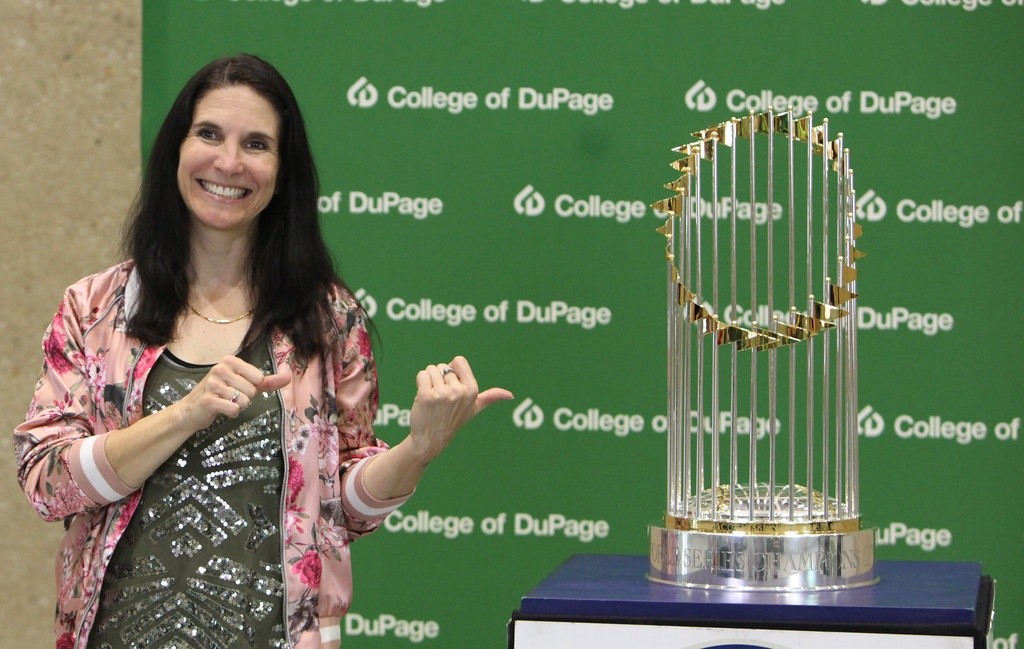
<box><xmin>10</xmin><ymin>55</ymin><xmax>516</xmax><ymax>649</ymax></box>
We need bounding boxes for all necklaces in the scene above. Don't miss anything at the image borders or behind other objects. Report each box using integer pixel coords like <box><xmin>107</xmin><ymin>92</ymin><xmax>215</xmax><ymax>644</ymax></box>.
<box><xmin>183</xmin><ymin>295</ymin><xmax>254</xmax><ymax>323</ymax></box>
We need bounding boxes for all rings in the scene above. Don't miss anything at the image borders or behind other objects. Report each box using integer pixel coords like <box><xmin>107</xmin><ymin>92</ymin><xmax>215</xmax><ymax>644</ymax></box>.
<box><xmin>441</xmin><ymin>368</ymin><xmax>456</xmax><ymax>375</ymax></box>
<box><xmin>229</xmin><ymin>390</ymin><xmax>240</xmax><ymax>402</ymax></box>
